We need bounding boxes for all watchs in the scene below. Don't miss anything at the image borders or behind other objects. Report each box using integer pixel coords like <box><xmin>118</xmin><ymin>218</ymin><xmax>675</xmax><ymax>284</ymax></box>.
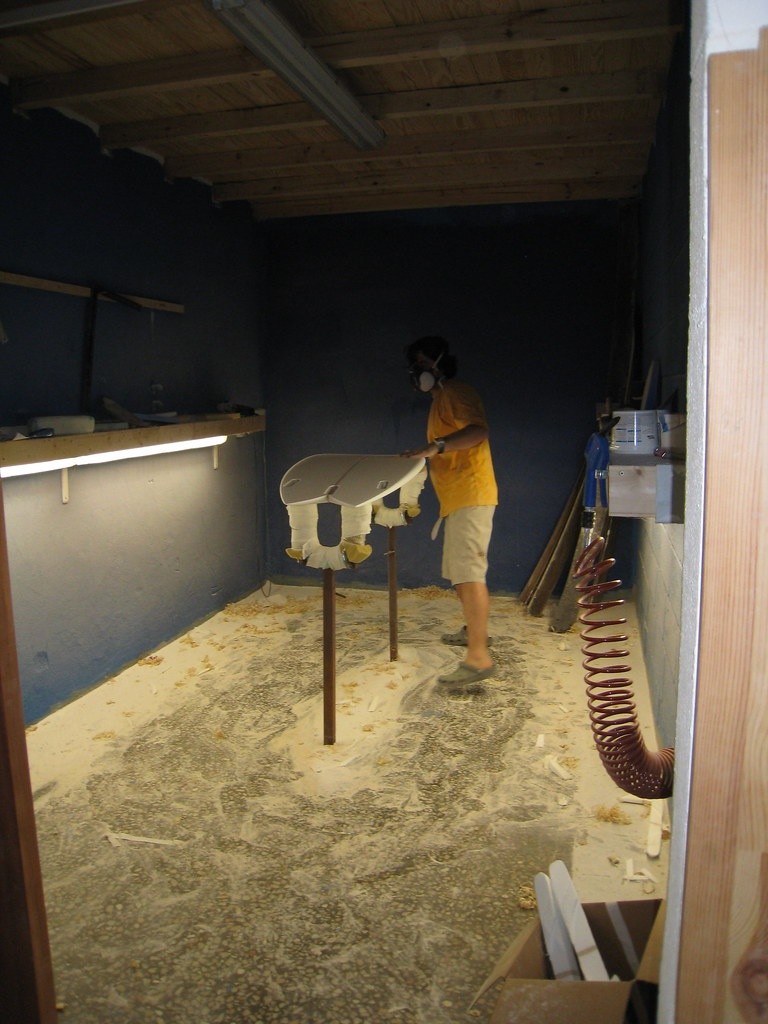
<box><xmin>432</xmin><ymin>437</ymin><xmax>445</xmax><ymax>454</ymax></box>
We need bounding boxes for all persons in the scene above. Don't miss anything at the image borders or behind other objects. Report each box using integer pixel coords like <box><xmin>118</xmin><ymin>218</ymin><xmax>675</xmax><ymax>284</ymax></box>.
<box><xmin>400</xmin><ymin>337</ymin><xmax>498</xmax><ymax>686</ymax></box>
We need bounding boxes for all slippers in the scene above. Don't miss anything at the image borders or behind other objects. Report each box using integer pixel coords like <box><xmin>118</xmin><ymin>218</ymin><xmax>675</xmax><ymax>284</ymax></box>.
<box><xmin>441</xmin><ymin>625</ymin><xmax>493</xmax><ymax>646</ymax></box>
<box><xmin>437</xmin><ymin>660</ymin><xmax>497</xmax><ymax>689</ymax></box>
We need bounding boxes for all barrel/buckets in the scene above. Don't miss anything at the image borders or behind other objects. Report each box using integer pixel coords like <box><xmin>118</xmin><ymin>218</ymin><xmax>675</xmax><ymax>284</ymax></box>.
<box><xmin>610</xmin><ymin>411</ymin><xmax>658</xmax><ymax>455</ymax></box>
